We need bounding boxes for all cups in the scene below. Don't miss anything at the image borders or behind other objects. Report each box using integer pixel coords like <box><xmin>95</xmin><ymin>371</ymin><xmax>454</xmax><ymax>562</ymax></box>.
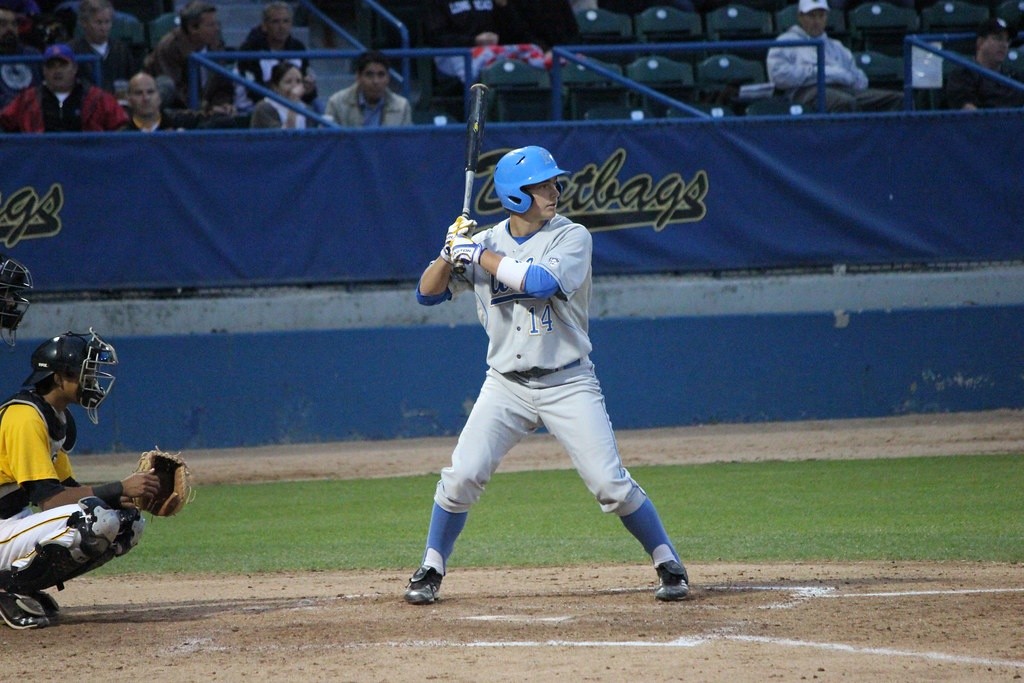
<box><xmin>114</xmin><ymin>81</ymin><xmax>130</xmax><ymax>106</ymax></box>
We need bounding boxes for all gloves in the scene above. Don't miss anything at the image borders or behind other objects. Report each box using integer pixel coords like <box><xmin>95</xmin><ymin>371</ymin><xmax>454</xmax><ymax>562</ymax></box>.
<box><xmin>449</xmin><ymin>233</ymin><xmax>487</xmax><ymax>265</ymax></box>
<box><xmin>439</xmin><ymin>216</ymin><xmax>478</xmax><ymax>265</ymax></box>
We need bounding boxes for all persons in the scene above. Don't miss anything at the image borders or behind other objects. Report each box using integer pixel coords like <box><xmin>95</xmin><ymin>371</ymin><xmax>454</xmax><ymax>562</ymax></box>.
<box><xmin>0</xmin><ymin>257</ymin><xmax>34</xmax><ymax>346</ymax></box>
<box><xmin>405</xmin><ymin>142</ymin><xmax>691</xmax><ymax>605</ymax></box>
<box><xmin>764</xmin><ymin>0</ymin><xmax>915</xmax><ymax>114</ymax></box>
<box><xmin>945</xmin><ymin>18</ymin><xmax>1024</xmax><ymax>111</ymax></box>
<box><xmin>0</xmin><ymin>0</ymin><xmax>410</xmax><ymax>133</ymax></box>
<box><xmin>313</xmin><ymin>0</ymin><xmax>582</xmax><ymax>123</ymax></box>
<box><xmin>0</xmin><ymin>326</ymin><xmax>160</xmax><ymax>631</ymax></box>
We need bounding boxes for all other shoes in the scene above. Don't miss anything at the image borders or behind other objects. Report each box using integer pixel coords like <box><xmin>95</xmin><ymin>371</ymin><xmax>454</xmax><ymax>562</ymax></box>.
<box><xmin>0</xmin><ymin>591</ymin><xmax>48</xmax><ymax>629</ymax></box>
<box><xmin>23</xmin><ymin>591</ymin><xmax>60</xmax><ymax>623</ymax></box>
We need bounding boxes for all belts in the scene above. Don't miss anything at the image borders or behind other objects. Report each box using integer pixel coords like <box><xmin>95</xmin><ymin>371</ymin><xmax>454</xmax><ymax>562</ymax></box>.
<box><xmin>512</xmin><ymin>358</ymin><xmax>580</xmax><ymax>379</ymax></box>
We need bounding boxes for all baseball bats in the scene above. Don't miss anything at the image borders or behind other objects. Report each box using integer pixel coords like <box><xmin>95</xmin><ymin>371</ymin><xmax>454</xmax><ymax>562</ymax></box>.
<box><xmin>453</xmin><ymin>83</ymin><xmax>492</xmax><ymax>274</ymax></box>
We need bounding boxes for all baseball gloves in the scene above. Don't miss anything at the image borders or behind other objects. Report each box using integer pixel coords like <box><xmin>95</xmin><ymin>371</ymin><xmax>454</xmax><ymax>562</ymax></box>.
<box><xmin>132</xmin><ymin>450</ymin><xmax>187</xmax><ymax>516</ymax></box>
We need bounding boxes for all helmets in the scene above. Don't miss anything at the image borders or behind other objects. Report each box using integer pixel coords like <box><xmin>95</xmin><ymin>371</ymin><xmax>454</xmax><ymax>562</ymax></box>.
<box><xmin>493</xmin><ymin>145</ymin><xmax>572</xmax><ymax>214</ymax></box>
<box><xmin>0</xmin><ymin>254</ymin><xmax>34</xmax><ymax>347</ymax></box>
<box><xmin>21</xmin><ymin>326</ymin><xmax>92</xmax><ymax>387</ymax></box>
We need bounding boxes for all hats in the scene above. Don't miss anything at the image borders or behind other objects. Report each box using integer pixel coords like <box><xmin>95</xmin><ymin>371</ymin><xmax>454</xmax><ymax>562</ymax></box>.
<box><xmin>798</xmin><ymin>0</ymin><xmax>830</xmax><ymax>14</ymax></box>
<box><xmin>977</xmin><ymin>17</ymin><xmax>1018</xmax><ymax>37</ymax></box>
<box><xmin>42</xmin><ymin>43</ymin><xmax>76</xmax><ymax>64</ymax></box>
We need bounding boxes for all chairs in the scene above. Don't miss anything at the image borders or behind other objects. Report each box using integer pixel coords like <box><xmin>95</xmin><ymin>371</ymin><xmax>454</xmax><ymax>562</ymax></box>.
<box><xmin>0</xmin><ymin>0</ymin><xmax>1024</xmax><ymax>120</ymax></box>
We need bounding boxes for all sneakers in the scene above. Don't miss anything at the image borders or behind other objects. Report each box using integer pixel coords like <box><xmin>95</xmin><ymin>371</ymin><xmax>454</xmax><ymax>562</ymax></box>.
<box><xmin>654</xmin><ymin>560</ymin><xmax>691</xmax><ymax>601</ymax></box>
<box><xmin>403</xmin><ymin>565</ymin><xmax>443</xmax><ymax>604</ymax></box>
